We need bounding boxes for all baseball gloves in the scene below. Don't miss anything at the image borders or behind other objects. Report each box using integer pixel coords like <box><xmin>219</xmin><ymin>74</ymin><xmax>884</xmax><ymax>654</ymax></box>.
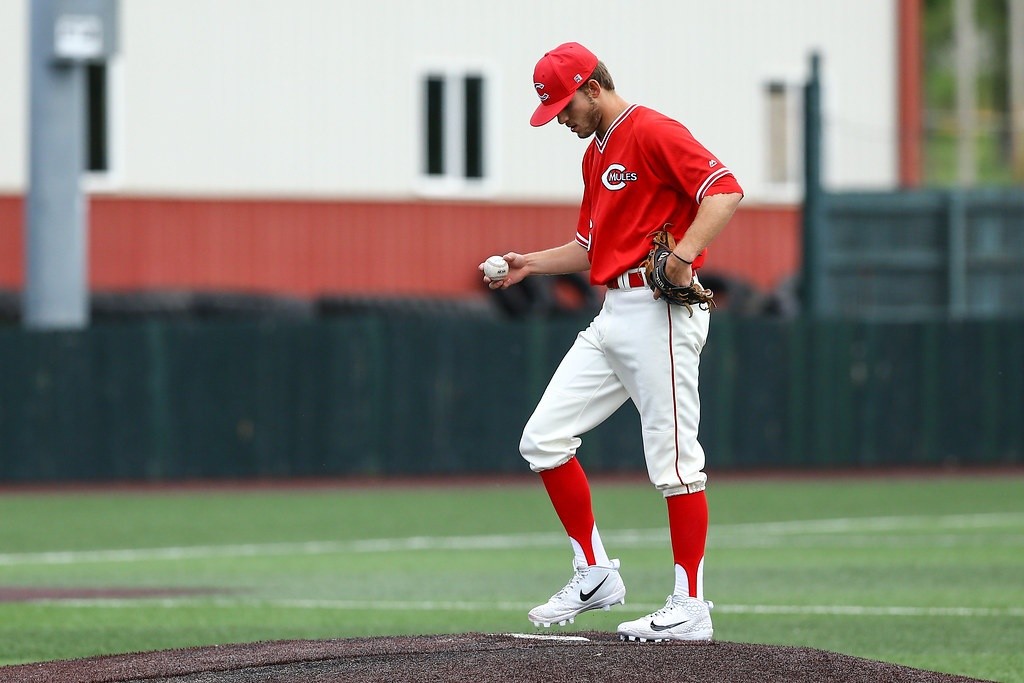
<box><xmin>644</xmin><ymin>231</ymin><xmax>714</xmax><ymax>307</ymax></box>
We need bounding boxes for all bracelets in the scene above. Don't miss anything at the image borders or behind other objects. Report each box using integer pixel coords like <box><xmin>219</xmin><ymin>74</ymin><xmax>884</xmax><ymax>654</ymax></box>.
<box><xmin>672</xmin><ymin>253</ymin><xmax>692</xmax><ymax>265</ymax></box>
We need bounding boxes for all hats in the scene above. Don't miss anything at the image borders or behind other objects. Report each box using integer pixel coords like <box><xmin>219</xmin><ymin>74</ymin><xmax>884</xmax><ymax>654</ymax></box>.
<box><xmin>530</xmin><ymin>42</ymin><xmax>598</xmax><ymax>127</ymax></box>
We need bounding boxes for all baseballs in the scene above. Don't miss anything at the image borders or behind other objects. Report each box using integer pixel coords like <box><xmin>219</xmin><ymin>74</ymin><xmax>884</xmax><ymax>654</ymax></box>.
<box><xmin>483</xmin><ymin>255</ymin><xmax>509</xmax><ymax>281</ymax></box>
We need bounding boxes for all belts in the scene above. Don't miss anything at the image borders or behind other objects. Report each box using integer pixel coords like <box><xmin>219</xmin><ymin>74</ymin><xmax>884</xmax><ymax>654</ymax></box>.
<box><xmin>605</xmin><ymin>269</ymin><xmax>695</xmax><ymax>290</ymax></box>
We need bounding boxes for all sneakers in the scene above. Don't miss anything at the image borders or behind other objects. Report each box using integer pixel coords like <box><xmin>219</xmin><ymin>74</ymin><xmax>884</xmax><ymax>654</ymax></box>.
<box><xmin>617</xmin><ymin>594</ymin><xmax>713</xmax><ymax>641</ymax></box>
<box><xmin>527</xmin><ymin>555</ymin><xmax>626</xmax><ymax>628</ymax></box>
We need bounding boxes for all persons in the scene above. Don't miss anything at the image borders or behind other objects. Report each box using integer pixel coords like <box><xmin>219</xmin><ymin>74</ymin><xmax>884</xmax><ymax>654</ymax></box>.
<box><xmin>478</xmin><ymin>42</ymin><xmax>743</xmax><ymax>642</ymax></box>
